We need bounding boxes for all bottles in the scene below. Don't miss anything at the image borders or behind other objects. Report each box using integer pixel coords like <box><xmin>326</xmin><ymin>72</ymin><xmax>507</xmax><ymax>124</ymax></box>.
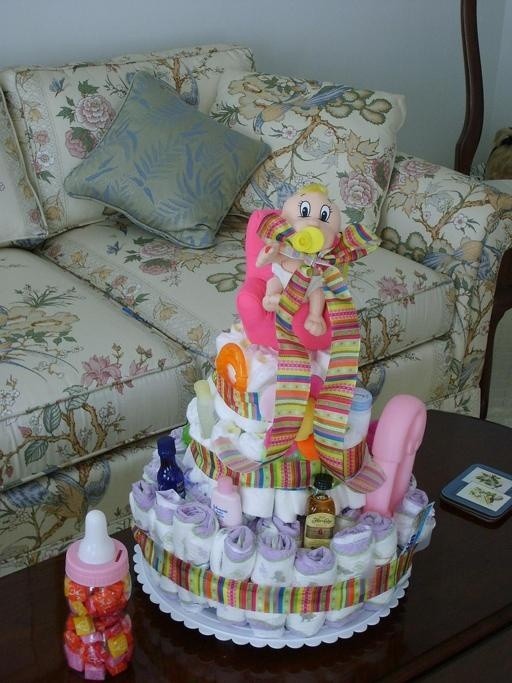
<box><xmin>60</xmin><ymin>508</ymin><xmax>136</xmax><ymax>680</ymax></box>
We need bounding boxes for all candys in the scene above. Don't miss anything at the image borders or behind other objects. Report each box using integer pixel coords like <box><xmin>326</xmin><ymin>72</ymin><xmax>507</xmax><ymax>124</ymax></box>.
<box><xmin>63</xmin><ymin>571</ymin><xmax>134</xmax><ymax>680</ymax></box>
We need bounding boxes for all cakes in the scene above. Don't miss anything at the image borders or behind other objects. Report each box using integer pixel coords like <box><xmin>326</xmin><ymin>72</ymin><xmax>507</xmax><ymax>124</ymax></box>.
<box><xmin>129</xmin><ymin>181</ymin><xmax>436</xmax><ymax>636</ymax></box>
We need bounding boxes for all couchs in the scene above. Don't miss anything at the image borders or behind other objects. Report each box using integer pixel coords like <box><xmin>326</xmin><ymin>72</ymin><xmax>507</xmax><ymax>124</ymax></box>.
<box><xmin>1</xmin><ymin>40</ymin><xmax>512</xmax><ymax>578</ymax></box>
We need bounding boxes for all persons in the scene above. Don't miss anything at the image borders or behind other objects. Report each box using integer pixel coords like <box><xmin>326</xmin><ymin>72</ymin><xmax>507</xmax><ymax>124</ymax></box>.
<box><xmin>251</xmin><ymin>182</ymin><xmax>342</xmax><ymax>337</ymax></box>
